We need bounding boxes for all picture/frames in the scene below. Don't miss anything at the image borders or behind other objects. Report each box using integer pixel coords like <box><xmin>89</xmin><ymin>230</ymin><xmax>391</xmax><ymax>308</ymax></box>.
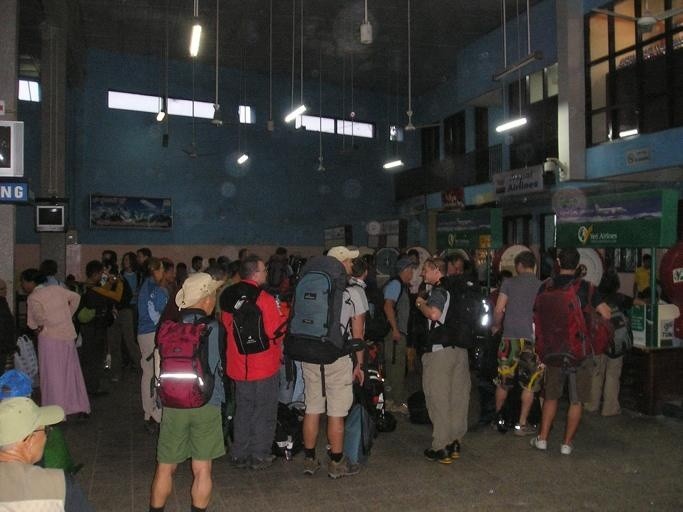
<box><xmin>89</xmin><ymin>192</ymin><xmax>174</xmax><ymax>229</ymax></box>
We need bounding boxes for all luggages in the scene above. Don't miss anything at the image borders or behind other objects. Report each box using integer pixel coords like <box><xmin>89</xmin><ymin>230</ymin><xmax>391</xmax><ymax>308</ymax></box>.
<box><xmin>84</xmin><ymin>316</ymin><xmax>114</xmax><ymax>393</ymax></box>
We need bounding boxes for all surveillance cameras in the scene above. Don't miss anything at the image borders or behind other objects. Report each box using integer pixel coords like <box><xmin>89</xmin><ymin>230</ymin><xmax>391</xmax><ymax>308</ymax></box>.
<box><xmin>544</xmin><ymin>157</ymin><xmax>566</xmax><ymax>176</ymax></box>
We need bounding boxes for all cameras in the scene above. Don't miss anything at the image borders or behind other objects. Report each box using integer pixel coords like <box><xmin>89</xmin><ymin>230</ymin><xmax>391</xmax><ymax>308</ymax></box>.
<box><xmin>112</xmin><ymin>276</ymin><xmax>117</xmax><ymax>281</ymax></box>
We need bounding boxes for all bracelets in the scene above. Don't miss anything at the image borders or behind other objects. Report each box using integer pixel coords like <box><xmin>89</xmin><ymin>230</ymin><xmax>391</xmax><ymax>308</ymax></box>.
<box><xmin>416</xmin><ymin>301</ymin><xmax>421</xmax><ymax>309</ymax></box>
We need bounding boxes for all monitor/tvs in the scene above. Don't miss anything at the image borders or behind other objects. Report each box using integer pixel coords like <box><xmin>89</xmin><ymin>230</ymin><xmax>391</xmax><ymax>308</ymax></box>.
<box><xmin>0</xmin><ymin>121</ymin><xmax>24</xmax><ymax>177</ymax></box>
<box><xmin>36</xmin><ymin>205</ymin><xmax>65</xmax><ymax>231</ymax></box>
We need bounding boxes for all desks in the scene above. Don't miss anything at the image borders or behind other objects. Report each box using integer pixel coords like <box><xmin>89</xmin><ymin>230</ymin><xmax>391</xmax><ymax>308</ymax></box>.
<box><xmin>623</xmin><ymin>335</ymin><xmax>683</xmax><ymax>418</ymax></box>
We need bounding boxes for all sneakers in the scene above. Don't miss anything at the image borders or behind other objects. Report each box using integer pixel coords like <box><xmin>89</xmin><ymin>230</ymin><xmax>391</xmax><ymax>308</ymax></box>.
<box><xmin>230</xmin><ymin>454</ymin><xmax>276</xmax><ymax>467</ymax></box>
<box><xmin>144</xmin><ymin>421</ymin><xmax>160</xmax><ymax>433</ymax></box>
<box><xmin>390</xmin><ymin>401</ymin><xmax>408</xmax><ymax>414</ymax></box>
<box><xmin>602</xmin><ymin>409</ymin><xmax>623</xmax><ymax>415</ymax></box>
<box><xmin>491</xmin><ymin>416</ymin><xmax>508</xmax><ymax>434</ymax></box>
<box><xmin>327</xmin><ymin>455</ymin><xmax>362</xmax><ymax>479</ymax></box>
<box><xmin>385</xmin><ymin>399</ymin><xmax>394</xmax><ymax>412</ymax></box>
<box><xmin>513</xmin><ymin>420</ymin><xmax>537</xmax><ymax>436</ymax></box>
<box><xmin>303</xmin><ymin>456</ymin><xmax>322</xmax><ymax>475</ymax></box>
<box><xmin>530</xmin><ymin>436</ymin><xmax>547</xmax><ymax>450</ymax></box>
<box><xmin>247</xmin><ymin>460</ymin><xmax>274</xmax><ymax>472</ymax></box>
<box><xmin>560</xmin><ymin>441</ymin><xmax>575</xmax><ymax>455</ymax></box>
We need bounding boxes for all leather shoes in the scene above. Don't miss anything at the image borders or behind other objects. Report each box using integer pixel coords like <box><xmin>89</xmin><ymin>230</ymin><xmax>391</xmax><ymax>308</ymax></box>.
<box><xmin>424</xmin><ymin>447</ymin><xmax>453</xmax><ymax>464</ymax></box>
<box><xmin>446</xmin><ymin>440</ymin><xmax>460</xmax><ymax>459</ymax></box>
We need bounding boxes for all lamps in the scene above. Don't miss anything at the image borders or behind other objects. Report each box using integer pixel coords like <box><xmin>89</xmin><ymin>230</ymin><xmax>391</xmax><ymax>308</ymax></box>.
<box><xmin>544</xmin><ymin>157</ymin><xmax>567</xmax><ymax>175</ymax></box>
<box><xmin>495</xmin><ymin>0</ymin><xmax>527</xmax><ymax>134</ymax></box>
<box><xmin>360</xmin><ymin>0</ymin><xmax>372</xmax><ymax>44</ymax></box>
<box><xmin>285</xmin><ymin>0</ymin><xmax>306</xmax><ymax>122</ymax></box>
<box><xmin>210</xmin><ymin>0</ymin><xmax>223</xmax><ymax>126</ymax></box>
<box><xmin>384</xmin><ymin>57</ymin><xmax>403</xmax><ymax>169</ymax></box>
<box><xmin>493</xmin><ymin>0</ymin><xmax>542</xmax><ymax>83</ymax></box>
<box><xmin>236</xmin><ymin>62</ymin><xmax>248</xmax><ymax>164</ymax></box>
<box><xmin>157</xmin><ymin>88</ymin><xmax>165</xmax><ymax>122</ymax></box>
<box><xmin>317</xmin><ymin>50</ymin><xmax>326</xmax><ymax>172</ymax></box>
<box><xmin>338</xmin><ymin>53</ymin><xmax>356</xmax><ymax>156</ymax></box>
<box><xmin>188</xmin><ymin>58</ymin><xmax>197</xmax><ymax>158</ymax></box>
<box><xmin>266</xmin><ymin>0</ymin><xmax>273</xmax><ymax>132</ymax></box>
<box><xmin>189</xmin><ymin>0</ymin><xmax>201</xmax><ymax>58</ymax></box>
<box><xmin>404</xmin><ymin>0</ymin><xmax>416</xmax><ymax>131</ymax></box>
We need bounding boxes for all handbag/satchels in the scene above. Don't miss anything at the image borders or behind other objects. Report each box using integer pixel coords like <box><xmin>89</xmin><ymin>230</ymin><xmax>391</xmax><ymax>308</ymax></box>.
<box><xmin>343</xmin><ymin>401</ymin><xmax>377</xmax><ymax>470</ymax></box>
<box><xmin>232</xmin><ymin>301</ymin><xmax>270</xmax><ymax>355</ymax></box>
<box><xmin>584</xmin><ymin>303</ymin><xmax>611</xmax><ymax>356</ymax></box>
<box><xmin>13</xmin><ymin>335</ymin><xmax>38</xmax><ymax>377</ymax></box>
<box><xmin>227</xmin><ymin>401</ymin><xmax>302</xmax><ymax>456</ymax></box>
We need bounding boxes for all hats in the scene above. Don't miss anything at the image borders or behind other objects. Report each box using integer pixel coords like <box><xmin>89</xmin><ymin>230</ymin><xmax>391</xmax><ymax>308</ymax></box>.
<box><xmin>327</xmin><ymin>245</ymin><xmax>359</xmax><ymax>262</ymax></box>
<box><xmin>0</xmin><ymin>370</ymin><xmax>33</xmax><ymax>399</ymax></box>
<box><xmin>175</xmin><ymin>272</ymin><xmax>224</xmax><ymax>309</ymax></box>
<box><xmin>396</xmin><ymin>258</ymin><xmax>416</xmax><ymax>271</ymax></box>
<box><xmin>0</xmin><ymin>397</ymin><xmax>65</xmax><ymax>447</ymax></box>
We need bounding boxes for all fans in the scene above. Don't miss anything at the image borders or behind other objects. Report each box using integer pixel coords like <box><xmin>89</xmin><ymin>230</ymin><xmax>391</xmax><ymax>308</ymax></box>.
<box><xmin>591</xmin><ymin>0</ymin><xmax>683</xmax><ymax>35</ymax></box>
<box><xmin>179</xmin><ymin>145</ymin><xmax>211</xmax><ymax>159</ymax></box>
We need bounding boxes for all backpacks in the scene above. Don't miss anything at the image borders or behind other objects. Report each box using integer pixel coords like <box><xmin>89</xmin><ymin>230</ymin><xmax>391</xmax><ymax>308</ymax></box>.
<box><xmin>426</xmin><ymin>275</ymin><xmax>479</xmax><ymax>348</ymax></box>
<box><xmin>282</xmin><ymin>254</ymin><xmax>353</xmax><ymax>365</ymax></box>
<box><xmin>606</xmin><ymin>304</ymin><xmax>633</xmax><ymax>358</ymax></box>
<box><xmin>354</xmin><ymin>368</ymin><xmax>386</xmax><ymax>414</ymax></box>
<box><xmin>155</xmin><ymin>315</ymin><xmax>216</xmax><ymax>409</ymax></box>
<box><xmin>365</xmin><ymin>277</ymin><xmax>402</xmax><ymax>343</ymax></box>
<box><xmin>532</xmin><ymin>277</ymin><xmax>591</xmax><ymax>368</ymax></box>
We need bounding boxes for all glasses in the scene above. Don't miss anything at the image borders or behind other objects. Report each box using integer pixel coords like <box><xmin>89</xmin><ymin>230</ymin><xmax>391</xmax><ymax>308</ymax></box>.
<box><xmin>252</xmin><ymin>267</ymin><xmax>269</xmax><ymax>273</ymax></box>
<box><xmin>424</xmin><ymin>258</ymin><xmax>440</xmax><ymax>271</ymax></box>
<box><xmin>24</xmin><ymin>427</ymin><xmax>53</xmax><ymax>444</ymax></box>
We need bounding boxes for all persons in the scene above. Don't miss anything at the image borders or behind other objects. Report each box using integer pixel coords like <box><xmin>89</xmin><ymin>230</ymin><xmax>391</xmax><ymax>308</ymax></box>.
<box><xmin>136</xmin><ymin>258</ymin><xmax>167</xmax><ymax>423</ymax></box>
<box><xmin>39</xmin><ymin>261</ymin><xmax>69</xmax><ymax>289</ymax></box>
<box><xmin>82</xmin><ymin>251</ymin><xmax>141</xmax><ymax>382</ymax></box>
<box><xmin>417</xmin><ymin>248</ymin><xmax>470</xmax><ymax>292</ymax></box>
<box><xmin>64</xmin><ymin>274</ymin><xmax>81</xmax><ymax>292</ymax></box>
<box><xmin>584</xmin><ymin>272</ymin><xmax>645</xmax><ymax>417</ymax></box>
<box><xmin>20</xmin><ymin>269</ymin><xmax>93</xmax><ymax>417</ymax></box>
<box><xmin>377</xmin><ymin>258</ymin><xmax>409</xmax><ymax>416</ymax></box>
<box><xmin>351</xmin><ymin>262</ymin><xmax>370</xmax><ymax>311</ymax></box>
<box><xmin>203</xmin><ymin>247</ymin><xmax>249</xmax><ymax>319</ymax></box>
<box><xmin>150</xmin><ymin>272</ymin><xmax>224</xmax><ymax>512</ymax></box>
<box><xmin>0</xmin><ymin>396</ymin><xmax>66</xmax><ymax>512</ymax></box>
<box><xmin>633</xmin><ymin>254</ymin><xmax>660</xmax><ymax>300</ymax></box>
<box><xmin>530</xmin><ymin>248</ymin><xmax>612</xmax><ymax>455</ymax></box>
<box><xmin>0</xmin><ymin>279</ymin><xmax>15</xmax><ymax>376</ymax></box>
<box><xmin>300</xmin><ymin>246</ymin><xmax>365</xmax><ymax>478</ymax></box>
<box><xmin>491</xmin><ymin>251</ymin><xmax>543</xmax><ymax>437</ymax></box>
<box><xmin>136</xmin><ymin>247</ymin><xmax>207</xmax><ymax>289</ymax></box>
<box><xmin>267</xmin><ymin>247</ymin><xmax>307</xmax><ymax>299</ymax></box>
<box><xmin>219</xmin><ymin>259</ymin><xmax>288</xmax><ymax>470</ymax></box>
<box><xmin>408</xmin><ymin>249</ymin><xmax>424</xmax><ymax>294</ymax></box>
<box><xmin>415</xmin><ymin>258</ymin><xmax>471</xmax><ymax>465</ymax></box>
